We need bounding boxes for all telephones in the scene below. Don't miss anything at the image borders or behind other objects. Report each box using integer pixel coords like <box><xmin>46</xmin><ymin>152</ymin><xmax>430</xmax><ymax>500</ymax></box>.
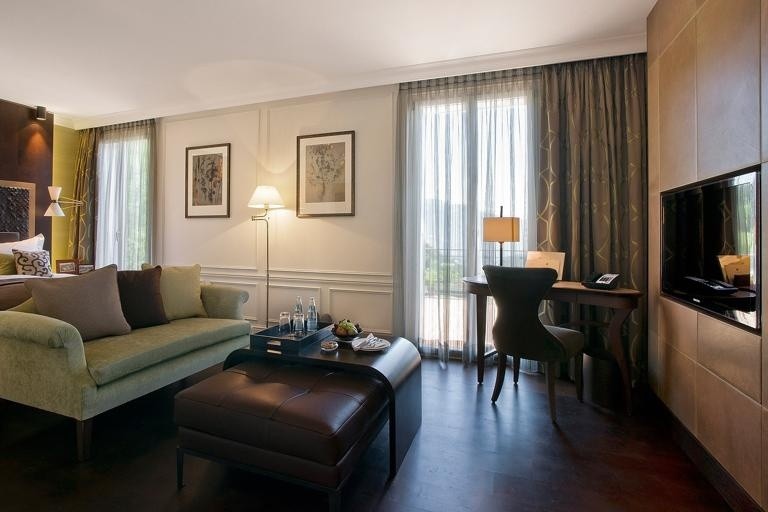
<box><xmin>673</xmin><ymin>275</ymin><xmax>739</xmax><ymax>296</ymax></box>
<box><xmin>581</xmin><ymin>271</ymin><xmax>621</xmax><ymax>289</ymax></box>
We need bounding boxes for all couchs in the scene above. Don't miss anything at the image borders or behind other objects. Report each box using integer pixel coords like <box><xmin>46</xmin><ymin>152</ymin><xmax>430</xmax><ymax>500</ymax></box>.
<box><xmin>0</xmin><ymin>283</ymin><xmax>252</xmax><ymax>467</ymax></box>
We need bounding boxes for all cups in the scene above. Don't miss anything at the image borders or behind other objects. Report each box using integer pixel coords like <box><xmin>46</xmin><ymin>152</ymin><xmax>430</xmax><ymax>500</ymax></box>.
<box><xmin>293</xmin><ymin>313</ymin><xmax>305</xmax><ymax>334</ymax></box>
<box><xmin>278</xmin><ymin>311</ymin><xmax>292</xmax><ymax>333</ymax></box>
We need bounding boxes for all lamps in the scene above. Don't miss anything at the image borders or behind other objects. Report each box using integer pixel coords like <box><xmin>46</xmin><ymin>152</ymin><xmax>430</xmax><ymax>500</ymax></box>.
<box><xmin>43</xmin><ymin>186</ymin><xmax>85</xmax><ymax>275</ymax></box>
<box><xmin>482</xmin><ymin>205</ymin><xmax>521</xmax><ymax>266</ymax></box>
<box><xmin>247</xmin><ymin>184</ymin><xmax>287</xmax><ymax>329</ymax></box>
<box><xmin>36</xmin><ymin>105</ymin><xmax>47</xmax><ymax>122</ymax></box>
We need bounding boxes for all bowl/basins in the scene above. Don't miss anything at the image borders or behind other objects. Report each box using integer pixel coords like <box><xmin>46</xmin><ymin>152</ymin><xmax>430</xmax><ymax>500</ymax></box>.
<box><xmin>331</xmin><ymin>329</ymin><xmax>364</xmax><ymax>341</ymax></box>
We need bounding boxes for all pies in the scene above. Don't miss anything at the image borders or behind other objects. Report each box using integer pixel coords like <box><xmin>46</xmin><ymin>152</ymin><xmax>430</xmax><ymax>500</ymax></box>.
<box><xmin>321</xmin><ymin>340</ymin><xmax>338</xmax><ymax>349</ymax></box>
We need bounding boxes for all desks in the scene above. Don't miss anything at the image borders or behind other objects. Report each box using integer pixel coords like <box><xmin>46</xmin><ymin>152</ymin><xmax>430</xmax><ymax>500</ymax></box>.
<box><xmin>460</xmin><ymin>275</ymin><xmax>644</xmax><ymax>418</ymax></box>
<box><xmin>662</xmin><ymin>283</ymin><xmax>757</xmax><ymax>311</ymax></box>
<box><xmin>222</xmin><ymin>331</ymin><xmax>423</xmax><ymax>485</ymax></box>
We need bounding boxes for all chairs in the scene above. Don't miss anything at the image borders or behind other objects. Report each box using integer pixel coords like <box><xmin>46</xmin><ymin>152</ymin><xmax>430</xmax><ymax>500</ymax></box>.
<box><xmin>482</xmin><ymin>263</ymin><xmax>587</xmax><ymax>424</ymax></box>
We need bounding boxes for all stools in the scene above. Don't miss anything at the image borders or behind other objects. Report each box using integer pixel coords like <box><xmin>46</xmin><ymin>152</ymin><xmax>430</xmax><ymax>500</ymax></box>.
<box><xmin>172</xmin><ymin>358</ymin><xmax>389</xmax><ymax>510</ymax></box>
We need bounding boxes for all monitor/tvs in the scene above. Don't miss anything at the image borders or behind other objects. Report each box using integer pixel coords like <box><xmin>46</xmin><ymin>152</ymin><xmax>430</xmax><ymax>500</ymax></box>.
<box><xmin>660</xmin><ymin>163</ymin><xmax>761</xmax><ymax>336</ymax></box>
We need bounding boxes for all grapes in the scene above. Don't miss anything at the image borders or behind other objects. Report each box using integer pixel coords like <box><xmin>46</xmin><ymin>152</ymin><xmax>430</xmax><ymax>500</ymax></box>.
<box><xmin>339</xmin><ymin>320</ymin><xmax>356</xmax><ymax>330</ymax></box>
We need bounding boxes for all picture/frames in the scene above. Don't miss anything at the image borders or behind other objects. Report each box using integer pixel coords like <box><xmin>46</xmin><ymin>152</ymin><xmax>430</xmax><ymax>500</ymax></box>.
<box><xmin>295</xmin><ymin>129</ymin><xmax>356</xmax><ymax>219</ymax></box>
<box><xmin>184</xmin><ymin>142</ymin><xmax>231</xmax><ymax>219</ymax></box>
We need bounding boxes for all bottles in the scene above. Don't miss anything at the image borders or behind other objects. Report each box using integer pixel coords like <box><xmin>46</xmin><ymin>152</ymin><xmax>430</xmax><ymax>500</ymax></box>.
<box><xmin>307</xmin><ymin>297</ymin><xmax>317</xmax><ymax>329</ymax></box>
<box><xmin>292</xmin><ymin>296</ymin><xmax>303</xmax><ymax>328</ymax></box>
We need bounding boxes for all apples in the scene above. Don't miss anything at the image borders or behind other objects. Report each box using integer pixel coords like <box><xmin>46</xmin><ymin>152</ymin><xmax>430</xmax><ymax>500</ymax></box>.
<box><xmin>348</xmin><ymin>328</ymin><xmax>357</xmax><ymax>335</ymax></box>
<box><xmin>335</xmin><ymin>327</ymin><xmax>346</xmax><ymax>334</ymax></box>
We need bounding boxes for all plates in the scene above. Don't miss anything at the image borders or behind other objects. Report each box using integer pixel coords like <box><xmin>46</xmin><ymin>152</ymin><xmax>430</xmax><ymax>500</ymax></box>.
<box><xmin>334</xmin><ymin>336</ymin><xmax>360</xmax><ymax>343</ymax></box>
<box><xmin>321</xmin><ymin>344</ymin><xmax>338</xmax><ymax>352</ymax></box>
<box><xmin>353</xmin><ymin>338</ymin><xmax>390</xmax><ymax>351</ymax></box>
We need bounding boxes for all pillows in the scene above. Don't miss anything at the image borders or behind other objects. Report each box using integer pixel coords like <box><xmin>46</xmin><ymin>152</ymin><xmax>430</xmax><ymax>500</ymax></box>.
<box><xmin>0</xmin><ymin>232</ymin><xmax>209</xmax><ymax>343</ymax></box>
